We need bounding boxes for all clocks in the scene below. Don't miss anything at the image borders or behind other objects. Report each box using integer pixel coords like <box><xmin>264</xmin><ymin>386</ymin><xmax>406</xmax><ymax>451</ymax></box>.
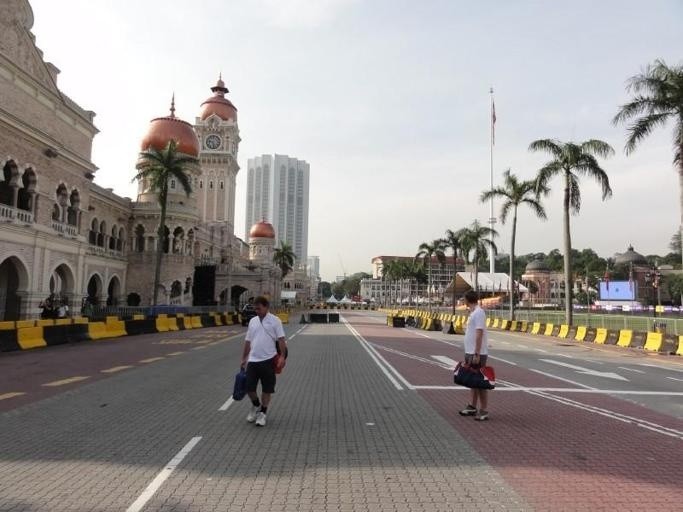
<box><xmin>203</xmin><ymin>133</ymin><xmax>222</xmax><ymax>151</ymax></box>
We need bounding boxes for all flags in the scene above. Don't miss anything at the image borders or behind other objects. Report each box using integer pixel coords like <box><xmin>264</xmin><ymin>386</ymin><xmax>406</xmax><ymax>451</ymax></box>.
<box><xmin>627</xmin><ymin>266</ymin><xmax>633</xmax><ymax>292</ymax></box>
<box><xmin>603</xmin><ymin>263</ymin><xmax>609</xmax><ymax>291</ymax></box>
<box><xmin>652</xmin><ymin>267</ymin><xmax>659</xmax><ymax>289</ymax></box>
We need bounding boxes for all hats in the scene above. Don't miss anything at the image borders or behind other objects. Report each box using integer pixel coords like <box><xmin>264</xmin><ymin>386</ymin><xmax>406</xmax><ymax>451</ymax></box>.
<box><xmin>273</xmin><ymin>354</ymin><xmax>286</xmax><ymax>374</ymax></box>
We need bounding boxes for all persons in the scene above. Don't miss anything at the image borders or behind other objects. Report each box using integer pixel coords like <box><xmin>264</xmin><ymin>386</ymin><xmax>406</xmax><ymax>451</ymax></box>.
<box><xmin>457</xmin><ymin>290</ymin><xmax>489</xmax><ymax>421</ymax></box>
<box><xmin>240</xmin><ymin>295</ymin><xmax>286</xmax><ymax>427</ymax></box>
<box><xmin>37</xmin><ymin>291</ymin><xmax>100</xmax><ymax>321</ymax></box>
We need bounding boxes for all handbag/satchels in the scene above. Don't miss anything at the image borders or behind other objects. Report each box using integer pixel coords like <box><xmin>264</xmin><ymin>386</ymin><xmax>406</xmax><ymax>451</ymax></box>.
<box><xmin>232</xmin><ymin>371</ymin><xmax>247</xmax><ymax>401</ymax></box>
<box><xmin>453</xmin><ymin>360</ymin><xmax>495</xmax><ymax>389</ymax></box>
<box><xmin>276</xmin><ymin>341</ymin><xmax>288</xmax><ymax>359</ymax></box>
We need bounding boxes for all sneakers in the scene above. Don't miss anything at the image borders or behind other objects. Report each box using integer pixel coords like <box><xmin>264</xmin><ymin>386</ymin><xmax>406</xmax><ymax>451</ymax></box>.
<box><xmin>475</xmin><ymin>409</ymin><xmax>489</xmax><ymax>422</ymax></box>
<box><xmin>247</xmin><ymin>404</ymin><xmax>261</xmax><ymax>423</ymax></box>
<box><xmin>459</xmin><ymin>405</ymin><xmax>477</xmax><ymax>416</ymax></box>
<box><xmin>255</xmin><ymin>409</ymin><xmax>266</xmax><ymax>426</ymax></box>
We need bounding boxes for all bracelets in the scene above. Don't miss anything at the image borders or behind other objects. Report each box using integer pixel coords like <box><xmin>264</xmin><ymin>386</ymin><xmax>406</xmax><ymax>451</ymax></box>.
<box><xmin>280</xmin><ymin>350</ymin><xmax>285</xmax><ymax>357</ymax></box>
<box><xmin>472</xmin><ymin>351</ymin><xmax>480</xmax><ymax>357</ymax></box>
